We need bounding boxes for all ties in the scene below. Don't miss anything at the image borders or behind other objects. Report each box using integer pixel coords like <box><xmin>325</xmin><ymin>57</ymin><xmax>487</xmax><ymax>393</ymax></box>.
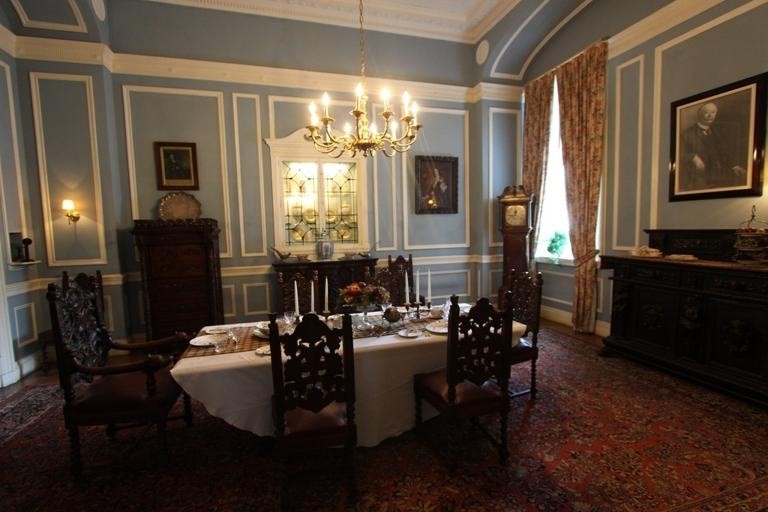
<box><xmin>696</xmin><ymin>124</ymin><xmax>713</xmax><ymax>136</ymax></box>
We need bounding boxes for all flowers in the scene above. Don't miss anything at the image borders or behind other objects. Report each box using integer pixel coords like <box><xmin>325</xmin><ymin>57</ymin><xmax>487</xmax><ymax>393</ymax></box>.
<box><xmin>338</xmin><ymin>281</ymin><xmax>389</xmax><ymax>313</ymax></box>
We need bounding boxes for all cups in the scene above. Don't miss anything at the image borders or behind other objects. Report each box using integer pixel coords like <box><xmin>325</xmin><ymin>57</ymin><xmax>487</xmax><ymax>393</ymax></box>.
<box><xmin>317</xmin><ymin>239</ymin><xmax>334</xmax><ymax>259</ymax></box>
<box><xmin>283</xmin><ymin>311</ymin><xmax>297</xmax><ymax>327</ymax></box>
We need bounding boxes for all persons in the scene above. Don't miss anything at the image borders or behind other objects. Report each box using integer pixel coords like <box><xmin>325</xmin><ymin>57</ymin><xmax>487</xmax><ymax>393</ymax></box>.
<box><xmin>679</xmin><ymin>101</ymin><xmax>747</xmax><ymax>190</ymax></box>
<box><xmin>421</xmin><ymin>167</ymin><xmax>450</xmax><ymax>210</ymax></box>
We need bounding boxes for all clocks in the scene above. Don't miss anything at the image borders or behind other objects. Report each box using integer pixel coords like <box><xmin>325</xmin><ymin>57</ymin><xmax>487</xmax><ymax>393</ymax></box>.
<box><xmin>496</xmin><ymin>183</ymin><xmax>538</xmax><ymax>313</ymax></box>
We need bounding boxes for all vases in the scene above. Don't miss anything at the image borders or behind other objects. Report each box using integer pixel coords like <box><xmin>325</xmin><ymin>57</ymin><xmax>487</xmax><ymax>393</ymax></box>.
<box><xmin>355</xmin><ymin>302</ymin><xmax>375</xmax><ymax>332</ymax></box>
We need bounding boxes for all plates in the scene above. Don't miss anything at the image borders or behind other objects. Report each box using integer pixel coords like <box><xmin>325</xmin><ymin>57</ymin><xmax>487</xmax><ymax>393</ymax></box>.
<box><xmin>253</xmin><ymin>329</ymin><xmax>268</xmax><ymax>338</ymax></box>
<box><xmin>425</xmin><ymin>320</ymin><xmax>448</xmax><ymax>333</ymax></box>
<box><xmin>255</xmin><ymin>319</ymin><xmax>272</xmax><ymax>332</ymax></box>
<box><xmin>157</xmin><ymin>192</ymin><xmax>202</xmax><ymax>222</ymax></box>
<box><xmin>394</xmin><ymin>306</ymin><xmax>412</xmax><ymax>314</ymax></box>
<box><xmin>255</xmin><ymin>343</ymin><xmax>271</xmax><ymax>355</ymax></box>
<box><xmin>397</xmin><ymin>328</ymin><xmax>423</xmax><ymax>338</ymax></box>
<box><xmin>664</xmin><ymin>255</ymin><xmax>697</xmax><ymax>261</ymax></box>
<box><xmin>631</xmin><ymin>252</ymin><xmax>661</xmax><ymax>258</ymax></box>
<box><xmin>203</xmin><ymin>324</ymin><xmax>232</xmax><ymax>334</ymax></box>
<box><xmin>190</xmin><ymin>333</ymin><xmax>229</xmax><ymax>348</ymax></box>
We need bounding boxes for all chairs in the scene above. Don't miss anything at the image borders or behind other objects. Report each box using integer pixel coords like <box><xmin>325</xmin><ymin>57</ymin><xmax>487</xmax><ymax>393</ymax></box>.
<box><xmin>264</xmin><ymin>310</ymin><xmax>361</xmax><ymax>510</ymax></box>
<box><xmin>498</xmin><ymin>266</ymin><xmax>545</xmax><ymax>405</ymax></box>
<box><xmin>410</xmin><ymin>289</ymin><xmax>514</xmax><ymax>479</ymax></box>
<box><xmin>276</xmin><ymin>269</ymin><xmax>321</xmax><ymax>316</ymax></box>
<box><xmin>378</xmin><ymin>253</ymin><xmax>415</xmax><ymax>307</ymax></box>
<box><xmin>45</xmin><ymin>268</ymin><xmax>193</xmax><ymax>479</ymax></box>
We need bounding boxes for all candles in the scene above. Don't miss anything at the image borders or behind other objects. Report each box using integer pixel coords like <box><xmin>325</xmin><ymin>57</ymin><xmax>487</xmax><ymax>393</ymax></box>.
<box><xmin>310</xmin><ymin>280</ymin><xmax>314</xmax><ymax>310</ymax></box>
<box><xmin>292</xmin><ymin>279</ymin><xmax>299</xmax><ymax>310</ymax></box>
<box><xmin>426</xmin><ymin>266</ymin><xmax>433</xmax><ymax>302</ymax></box>
<box><xmin>403</xmin><ymin>269</ymin><xmax>409</xmax><ymax>305</ymax></box>
<box><xmin>414</xmin><ymin>268</ymin><xmax>421</xmax><ymax>301</ymax></box>
<box><xmin>324</xmin><ymin>277</ymin><xmax>329</xmax><ymax>311</ymax></box>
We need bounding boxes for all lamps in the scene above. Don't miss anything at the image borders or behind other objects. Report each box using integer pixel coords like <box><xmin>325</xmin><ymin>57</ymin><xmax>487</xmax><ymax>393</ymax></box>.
<box><xmin>62</xmin><ymin>199</ymin><xmax>82</xmax><ymax>224</ymax></box>
<box><xmin>304</xmin><ymin>1</ymin><xmax>422</xmax><ymax>160</ymax></box>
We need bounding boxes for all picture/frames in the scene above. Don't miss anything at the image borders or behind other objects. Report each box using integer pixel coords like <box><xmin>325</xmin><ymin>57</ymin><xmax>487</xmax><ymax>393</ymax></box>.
<box><xmin>153</xmin><ymin>138</ymin><xmax>200</xmax><ymax>192</ymax></box>
<box><xmin>414</xmin><ymin>154</ymin><xmax>461</xmax><ymax>216</ymax></box>
<box><xmin>667</xmin><ymin>68</ymin><xmax>768</xmax><ymax>206</ymax></box>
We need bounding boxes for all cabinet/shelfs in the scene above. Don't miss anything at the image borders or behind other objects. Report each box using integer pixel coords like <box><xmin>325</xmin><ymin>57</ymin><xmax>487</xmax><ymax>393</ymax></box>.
<box><xmin>270</xmin><ymin>254</ymin><xmax>382</xmax><ymax>315</ymax></box>
<box><xmin>595</xmin><ymin>226</ymin><xmax>767</xmax><ymax>411</ymax></box>
<box><xmin>129</xmin><ymin>216</ymin><xmax>226</xmax><ymax>341</ymax></box>
